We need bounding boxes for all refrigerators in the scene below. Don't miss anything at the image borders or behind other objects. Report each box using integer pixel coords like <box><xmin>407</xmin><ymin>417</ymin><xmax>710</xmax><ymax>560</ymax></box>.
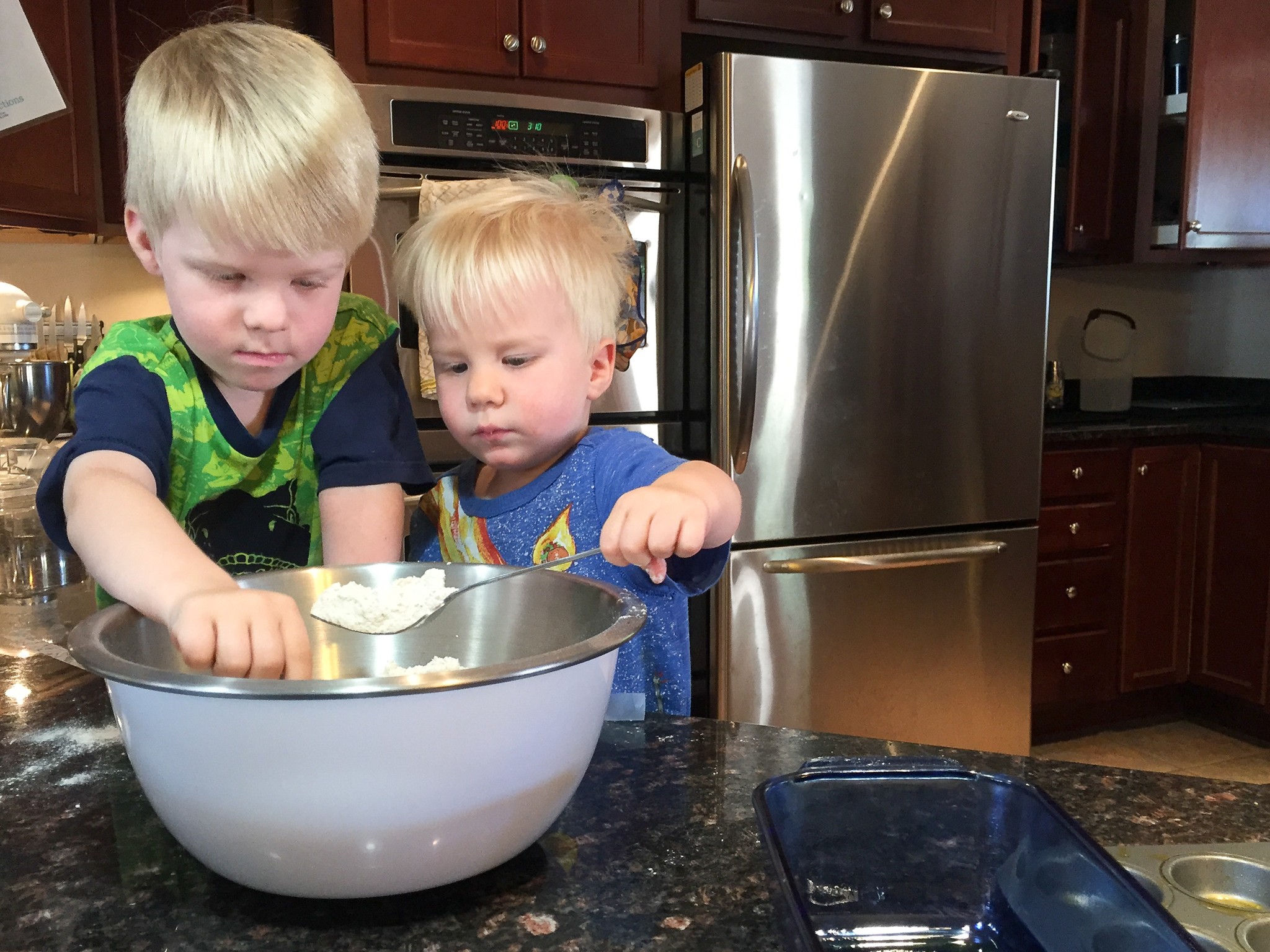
<box><xmin>682</xmin><ymin>50</ymin><xmax>1062</xmax><ymax>760</ymax></box>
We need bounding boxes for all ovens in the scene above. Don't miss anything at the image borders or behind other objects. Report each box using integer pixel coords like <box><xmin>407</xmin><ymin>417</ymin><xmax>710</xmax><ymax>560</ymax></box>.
<box><xmin>350</xmin><ymin>81</ymin><xmax>683</xmax><ymax>560</ymax></box>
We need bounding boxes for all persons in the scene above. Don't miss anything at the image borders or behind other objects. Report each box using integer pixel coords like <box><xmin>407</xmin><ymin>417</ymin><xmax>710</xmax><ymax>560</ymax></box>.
<box><xmin>395</xmin><ymin>178</ymin><xmax>742</xmax><ymax>717</ymax></box>
<box><xmin>36</xmin><ymin>24</ymin><xmax>435</xmax><ymax>680</ymax></box>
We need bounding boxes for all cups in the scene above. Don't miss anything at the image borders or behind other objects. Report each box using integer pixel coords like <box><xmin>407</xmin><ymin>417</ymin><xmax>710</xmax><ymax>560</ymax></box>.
<box><xmin>1045</xmin><ymin>360</ymin><xmax>1064</xmax><ymax>410</ymax></box>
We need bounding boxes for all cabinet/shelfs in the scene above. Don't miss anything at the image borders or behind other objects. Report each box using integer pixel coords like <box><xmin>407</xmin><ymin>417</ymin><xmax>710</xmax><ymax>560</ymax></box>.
<box><xmin>1030</xmin><ymin>435</ymin><xmax>1270</xmax><ymax>749</ymax></box>
<box><xmin>0</xmin><ymin>0</ymin><xmax>1270</xmax><ymax>263</ymax></box>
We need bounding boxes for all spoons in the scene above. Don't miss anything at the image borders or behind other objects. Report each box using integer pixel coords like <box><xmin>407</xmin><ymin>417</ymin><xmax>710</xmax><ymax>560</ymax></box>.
<box><xmin>310</xmin><ymin>549</ymin><xmax>602</xmax><ymax>635</ymax></box>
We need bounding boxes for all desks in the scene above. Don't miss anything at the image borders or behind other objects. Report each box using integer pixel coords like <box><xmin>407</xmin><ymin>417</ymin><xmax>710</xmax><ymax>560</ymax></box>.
<box><xmin>0</xmin><ymin>581</ymin><xmax>1270</xmax><ymax>952</ymax></box>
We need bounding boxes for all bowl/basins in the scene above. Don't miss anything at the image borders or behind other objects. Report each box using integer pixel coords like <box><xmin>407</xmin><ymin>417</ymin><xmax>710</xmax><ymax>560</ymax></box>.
<box><xmin>0</xmin><ymin>515</ymin><xmax>88</xmax><ymax>592</ymax></box>
<box><xmin>1</xmin><ymin>360</ymin><xmax>72</xmax><ymax>444</ymax></box>
<box><xmin>67</xmin><ymin>559</ymin><xmax>655</xmax><ymax>898</ymax></box>
